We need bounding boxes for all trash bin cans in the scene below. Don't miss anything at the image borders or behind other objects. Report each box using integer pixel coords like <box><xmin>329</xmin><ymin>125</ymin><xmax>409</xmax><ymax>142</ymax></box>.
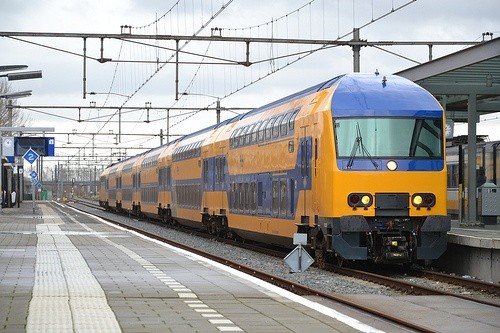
<box><xmin>478</xmin><ymin>180</ymin><xmax>500</xmax><ymax>217</ymax></box>
<box><xmin>39</xmin><ymin>190</ymin><xmax>47</xmax><ymax>200</ymax></box>
<box><xmin>47</xmin><ymin>190</ymin><xmax>53</xmax><ymax>201</ymax></box>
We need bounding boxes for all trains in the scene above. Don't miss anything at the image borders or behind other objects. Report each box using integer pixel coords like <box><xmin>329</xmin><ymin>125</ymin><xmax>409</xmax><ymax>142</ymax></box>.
<box><xmin>97</xmin><ymin>68</ymin><xmax>449</xmax><ymax>270</ymax></box>
